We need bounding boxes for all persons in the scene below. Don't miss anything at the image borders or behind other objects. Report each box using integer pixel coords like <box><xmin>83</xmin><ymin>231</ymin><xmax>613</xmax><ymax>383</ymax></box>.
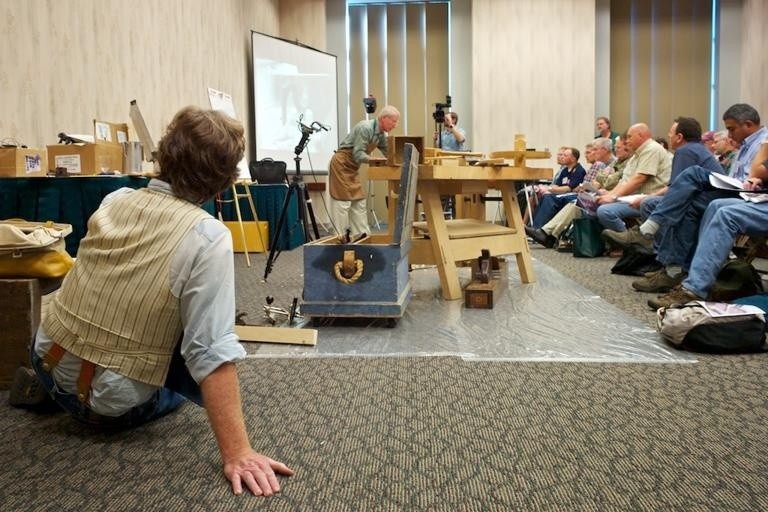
<box><xmin>432</xmin><ymin>112</ymin><xmax>467</xmax><ymax>152</ymax></box>
<box><xmin>325</xmin><ymin>105</ymin><xmax>400</xmax><ymax>238</ymax></box>
<box><xmin>8</xmin><ymin>105</ymin><xmax>295</xmax><ymax>496</ymax></box>
<box><xmin>517</xmin><ymin>103</ymin><xmax>767</xmax><ymax>310</ymax></box>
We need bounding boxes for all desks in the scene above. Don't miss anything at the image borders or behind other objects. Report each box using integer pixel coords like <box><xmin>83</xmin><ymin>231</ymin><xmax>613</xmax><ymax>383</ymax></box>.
<box><xmin>1</xmin><ymin>177</ymin><xmax>305</xmax><ymax>256</ymax></box>
<box><xmin>365</xmin><ymin>133</ymin><xmax>553</xmax><ymax>300</ymax></box>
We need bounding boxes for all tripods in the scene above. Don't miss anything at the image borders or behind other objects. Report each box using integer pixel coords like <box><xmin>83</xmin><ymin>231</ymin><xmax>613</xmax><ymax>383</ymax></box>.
<box><xmin>262</xmin><ymin>113</ymin><xmax>329</xmax><ymax>286</ymax></box>
<box><xmin>366</xmin><ymin>114</ymin><xmax>383</xmax><ymax>231</ymax></box>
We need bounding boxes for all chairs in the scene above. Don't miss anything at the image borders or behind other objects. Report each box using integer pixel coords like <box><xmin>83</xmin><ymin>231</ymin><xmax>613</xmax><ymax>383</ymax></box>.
<box><xmin>731</xmin><ymin>233</ymin><xmax>768</xmax><ymax>267</ymax></box>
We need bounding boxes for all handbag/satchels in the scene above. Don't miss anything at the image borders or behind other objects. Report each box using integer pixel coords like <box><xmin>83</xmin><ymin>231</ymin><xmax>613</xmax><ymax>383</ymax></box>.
<box><xmin>249</xmin><ymin>157</ymin><xmax>286</xmax><ymax>183</ymax></box>
<box><xmin>0</xmin><ymin>217</ymin><xmax>74</xmax><ymax>278</ymax></box>
<box><xmin>712</xmin><ymin>257</ymin><xmax>764</xmax><ymax>298</ymax></box>
<box><xmin>570</xmin><ymin>211</ymin><xmax>604</xmax><ymax>258</ymax></box>
<box><xmin>656</xmin><ymin>294</ymin><xmax>767</xmax><ymax>353</ymax></box>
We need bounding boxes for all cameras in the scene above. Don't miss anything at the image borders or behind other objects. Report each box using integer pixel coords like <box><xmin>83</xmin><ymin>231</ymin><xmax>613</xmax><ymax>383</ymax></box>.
<box><xmin>432</xmin><ymin>95</ymin><xmax>452</xmax><ymax>123</ymax></box>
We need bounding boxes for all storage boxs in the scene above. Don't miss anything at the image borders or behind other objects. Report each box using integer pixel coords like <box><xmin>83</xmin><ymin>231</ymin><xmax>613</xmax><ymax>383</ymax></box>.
<box><xmin>463</xmin><ymin>279</ymin><xmax>500</xmax><ymax>309</ymax></box>
<box><xmin>470</xmin><ymin>258</ymin><xmax>508</xmax><ymax>297</ymax></box>
<box><xmin>0</xmin><ymin>145</ymin><xmax>46</xmax><ymax>179</ymax></box>
<box><xmin>300</xmin><ymin>145</ymin><xmax>419</xmax><ymax>328</ymax></box>
<box><xmin>125</xmin><ymin>141</ymin><xmax>144</xmax><ymax>176</ymax></box>
<box><xmin>46</xmin><ymin>142</ymin><xmax>123</xmax><ymax>175</ymax></box>
<box><xmin>0</xmin><ymin>277</ymin><xmax>41</xmax><ymax>393</ymax></box>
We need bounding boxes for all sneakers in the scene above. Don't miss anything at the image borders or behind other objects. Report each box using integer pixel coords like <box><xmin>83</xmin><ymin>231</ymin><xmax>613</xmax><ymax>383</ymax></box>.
<box><xmin>8</xmin><ymin>365</ymin><xmax>50</xmax><ymax>408</ymax></box>
<box><xmin>602</xmin><ymin>224</ymin><xmax>703</xmax><ymax>309</ymax></box>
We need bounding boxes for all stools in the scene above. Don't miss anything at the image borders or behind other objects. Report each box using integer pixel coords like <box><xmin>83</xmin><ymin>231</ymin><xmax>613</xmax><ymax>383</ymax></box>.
<box><xmin>223</xmin><ymin>220</ymin><xmax>269</xmax><ymax>253</ymax></box>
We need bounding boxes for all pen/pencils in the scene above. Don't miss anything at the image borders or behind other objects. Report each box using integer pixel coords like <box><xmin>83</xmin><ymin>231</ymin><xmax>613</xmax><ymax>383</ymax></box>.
<box><xmin>747</xmin><ymin>180</ymin><xmax>764</xmax><ymax>187</ymax></box>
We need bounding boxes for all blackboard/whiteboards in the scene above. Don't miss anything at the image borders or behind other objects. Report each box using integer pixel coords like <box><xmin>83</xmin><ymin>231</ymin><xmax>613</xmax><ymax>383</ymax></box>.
<box><xmin>249</xmin><ymin>30</ymin><xmax>340</xmax><ymax>175</ymax></box>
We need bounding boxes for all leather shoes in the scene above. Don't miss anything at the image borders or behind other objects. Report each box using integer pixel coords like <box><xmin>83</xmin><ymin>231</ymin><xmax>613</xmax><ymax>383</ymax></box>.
<box><xmin>524</xmin><ymin>224</ymin><xmax>556</xmax><ymax>250</ymax></box>
